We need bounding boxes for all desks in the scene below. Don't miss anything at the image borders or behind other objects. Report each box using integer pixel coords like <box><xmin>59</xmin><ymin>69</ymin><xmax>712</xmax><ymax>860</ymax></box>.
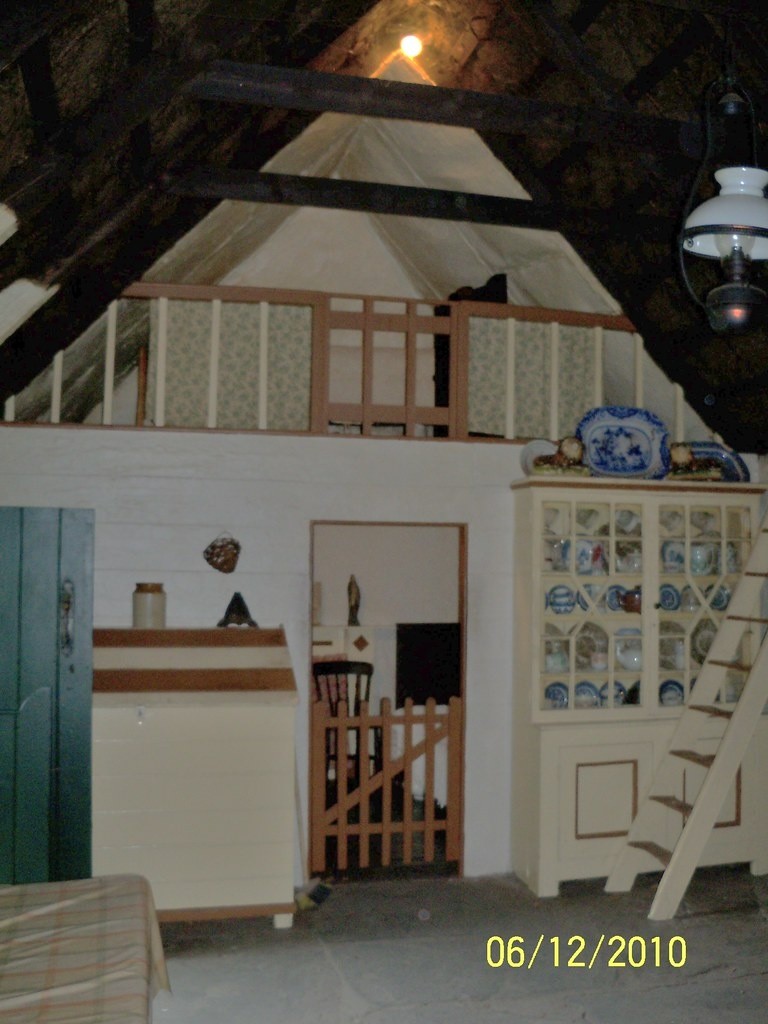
<box><xmin>0</xmin><ymin>873</ymin><xmax>153</xmax><ymax>1024</ymax></box>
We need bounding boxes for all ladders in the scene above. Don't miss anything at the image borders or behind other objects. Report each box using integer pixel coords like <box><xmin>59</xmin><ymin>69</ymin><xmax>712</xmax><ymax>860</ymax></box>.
<box><xmin>604</xmin><ymin>511</ymin><xmax>768</xmax><ymax>918</ymax></box>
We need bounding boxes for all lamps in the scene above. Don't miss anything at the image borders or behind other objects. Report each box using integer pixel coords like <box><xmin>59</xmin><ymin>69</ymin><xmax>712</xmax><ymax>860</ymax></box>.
<box><xmin>678</xmin><ymin>14</ymin><xmax>768</xmax><ymax>337</ymax></box>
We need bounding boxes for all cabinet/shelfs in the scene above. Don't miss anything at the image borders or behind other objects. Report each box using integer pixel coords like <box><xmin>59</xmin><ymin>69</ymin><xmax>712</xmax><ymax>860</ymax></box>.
<box><xmin>511</xmin><ymin>475</ymin><xmax>768</xmax><ymax>899</ymax></box>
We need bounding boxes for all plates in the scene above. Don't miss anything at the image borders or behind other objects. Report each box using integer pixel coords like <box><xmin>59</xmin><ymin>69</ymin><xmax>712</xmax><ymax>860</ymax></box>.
<box><xmin>520</xmin><ymin>438</ymin><xmax>559</xmax><ymax>478</ymax></box>
<box><xmin>544</xmin><ymin>539</ymin><xmax>732</xmax><ymax>711</ymax></box>
<box><xmin>575</xmin><ymin>404</ymin><xmax>750</xmax><ymax>483</ymax></box>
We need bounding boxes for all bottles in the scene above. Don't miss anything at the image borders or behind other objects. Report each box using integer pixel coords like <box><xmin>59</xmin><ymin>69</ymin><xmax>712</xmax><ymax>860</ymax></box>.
<box><xmin>131</xmin><ymin>582</ymin><xmax>167</xmax><ymax>631</ymax></box>
